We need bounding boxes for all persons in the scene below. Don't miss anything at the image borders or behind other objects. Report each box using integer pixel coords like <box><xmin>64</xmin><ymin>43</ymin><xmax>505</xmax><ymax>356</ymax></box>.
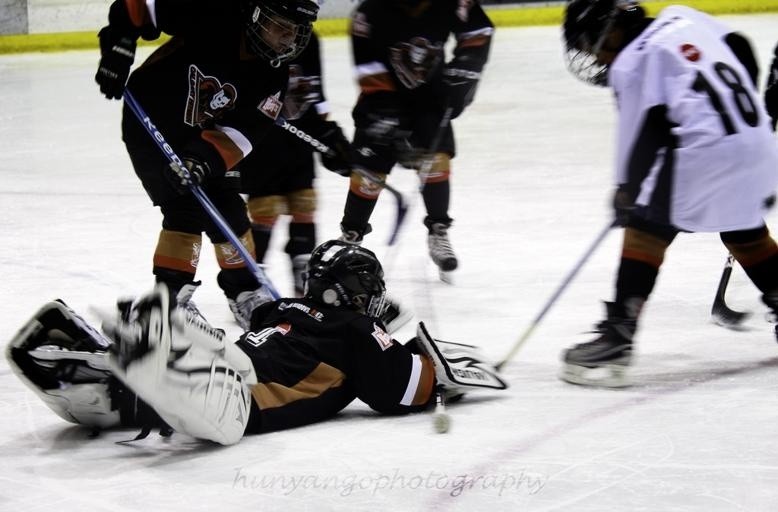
<box><xmin>766</xmin><ymin>41</ymin><xmax>778</xmax><ymax>135</ymax></box>
<box><xmin>559</xmin><ymin>1</ymin><xmax>778</xmax><ymax>367</ymax></box>
<box><xmin>245</xmin><ymin>32</ymin><xmax>359</xmax><ymax>293</ymax></box>
<box><xmin>8</xmin><ymin>240</ymin><xmax>508</xmax><ymax>447</ymax></box>
<box><xmin>334</xmin><ymin>1</ymin><xmax>493</xmax><ymax>269</ymax></box>
<box><xmin>95</xmin><ymin>0</ymin><xmax>320</xmax><ymax>330</ymax></box>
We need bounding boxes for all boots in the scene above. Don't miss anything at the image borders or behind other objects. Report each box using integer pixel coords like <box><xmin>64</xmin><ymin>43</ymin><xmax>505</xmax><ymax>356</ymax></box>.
<box><xmin>337</xmin><ymin>225</ymin><xmax>366</xmax><ymax>248</ymax></box>
<box><xmin>560</xmin><ymin>308</ymin><xmax>640</xmax><ymax>369</ymax></box>
<box><xmin>427</xmin><ymin>223</ymin><xmax>459</xmax><ymax>270</ymax></box>
<box><xmin>158</xmin><ymin>280</ymin><xmax>229</xmax><ymax>339</ymax></box>
<box><xmin>215</xmin><ymin>268</ymin><xmax>276</xmax><ymax>330</ymax></box>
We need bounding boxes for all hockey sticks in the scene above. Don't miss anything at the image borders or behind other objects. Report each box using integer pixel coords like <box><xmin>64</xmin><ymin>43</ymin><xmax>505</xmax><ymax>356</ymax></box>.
<box><xmin>388</xmin><ymin>106</ymin><xmax>454</xmax><ymax>245</ymax></box>
<box><xmin>273</xmin><ymin>116</ymin><xmax>401</xmax><ymax>223</ymax></box>
<box><xmin>447</xmin><ymin>217</ymin><xmax>615</xmax><ymax>403</ymax></box>
<box><xmin>712</xmin><ymin>118</ymin><xmax>777</xmax><ymax>327</ymax></box>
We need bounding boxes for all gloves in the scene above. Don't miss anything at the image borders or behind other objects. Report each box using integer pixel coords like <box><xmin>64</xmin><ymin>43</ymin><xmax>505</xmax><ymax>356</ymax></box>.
<box><xmin>317</xmin><ymin>139</ymin><xmax>362</xmax><ymax>180</ymax></box>
<box><xmin>167</xmin><ymin>154</ymin><xmax>215</xmax><ymax>193</ymax></box>
<box><xmin>609</xmin><ymin>183</ymin><xmax>642</xmax><ymax>228</ymax></box>
<box><xmin>432</xmin><ymin>76</ymin><xmax>478</xmax><ymax>120</ymax></box>
<box><xmin>94</xmin><ymin>32</ymin><xmax>133</xmax><ymax>101</ymax></box>
<box><xmin>395</xmin><ymin>133</ymin><xmax>431</xmax><ymax>170</ymax></box>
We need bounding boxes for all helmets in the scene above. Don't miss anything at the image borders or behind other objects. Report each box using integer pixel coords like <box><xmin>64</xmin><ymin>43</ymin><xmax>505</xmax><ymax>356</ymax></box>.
<box><xmin>305</xmin><ymin>239</ymin><xmax>387</xmax><ymax>321</ymax></box>
<box><xmin>248</xmin><ymin>0</ymin><xmax>321</xmax><ymax>68</ymax></box>
<box><xmin>563</xmin><ymin>0</ymin><xmax>643</xmax><ymax>87</ymax></box>
